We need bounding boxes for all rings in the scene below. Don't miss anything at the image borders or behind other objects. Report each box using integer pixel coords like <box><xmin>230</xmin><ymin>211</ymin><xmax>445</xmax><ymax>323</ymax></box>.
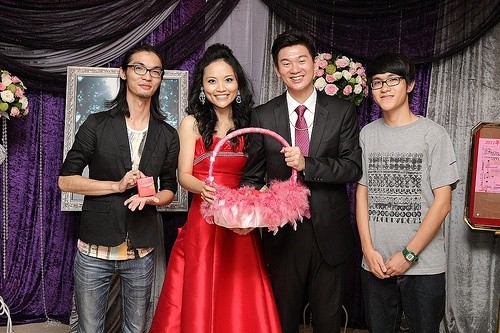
<box><xmin>133</xmin><ymin>174</ymin><xmax>136</xmax><ymax>176</ymax></box>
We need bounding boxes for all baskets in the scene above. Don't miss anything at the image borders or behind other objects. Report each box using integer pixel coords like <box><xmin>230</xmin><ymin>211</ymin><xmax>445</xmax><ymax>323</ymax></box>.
<box><xmin>201</xmin><ymin>128</ymin><xmax>311</xmax><ymax>234</ymax></box>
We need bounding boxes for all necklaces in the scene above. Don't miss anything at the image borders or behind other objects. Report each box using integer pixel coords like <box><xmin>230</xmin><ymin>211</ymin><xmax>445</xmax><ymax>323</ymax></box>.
<box><xmin>291</xmin><ymin>121</ymin><xmax>313</xmax><ymax>131</ymax></box>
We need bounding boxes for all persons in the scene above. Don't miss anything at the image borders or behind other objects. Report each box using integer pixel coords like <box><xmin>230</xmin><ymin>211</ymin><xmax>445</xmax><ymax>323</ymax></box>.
<box><xmin>148</xmin><ymin>42</ymin><xmax>284</xmax><ymax>333</ymax></box>
<box><xmin>239</xmin><ymin>30</ymin><xmax>362</xmax><ymax>333</ymax></box>
<box><xmin>58</xmin><ymin>44</ymin><xmax>181</xmax><ymax>333</ymax></box>
<box><xmin>356</xmin><ymin>53</ymin><xmax>460</xmax><ymax>333</ymax></box>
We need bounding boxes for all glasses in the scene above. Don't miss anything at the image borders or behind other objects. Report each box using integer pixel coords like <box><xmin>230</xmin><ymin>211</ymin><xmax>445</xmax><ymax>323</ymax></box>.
<box><xmin>370</xmin><ymin>76</ymin><xmax>405</xmax><ymax>90</ymax></box>
<box><xmin>126</xmin><ymin>63</ymin><xmax>163</xmax><ymax>78</ymax></box>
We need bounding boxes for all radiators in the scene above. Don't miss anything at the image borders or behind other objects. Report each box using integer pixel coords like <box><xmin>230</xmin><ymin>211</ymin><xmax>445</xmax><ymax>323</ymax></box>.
<box><xmin>0</xmin><ymin>70</ymin><xmax>29</xmax><ymax>120</ymax></box>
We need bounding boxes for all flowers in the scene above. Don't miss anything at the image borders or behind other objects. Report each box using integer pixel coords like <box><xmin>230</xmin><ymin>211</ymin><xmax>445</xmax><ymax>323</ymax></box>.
<box><xmin>312</xmin><ymin>52</ymin><xmax>369</xmax><ymax>106</ymax></box>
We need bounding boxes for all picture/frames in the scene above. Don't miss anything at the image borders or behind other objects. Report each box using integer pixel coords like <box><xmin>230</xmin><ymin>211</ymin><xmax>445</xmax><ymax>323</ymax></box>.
<box><xmin>60</xmin><ymin>66</ymin><xmax>189</xmax><ymax>211</ymax></box>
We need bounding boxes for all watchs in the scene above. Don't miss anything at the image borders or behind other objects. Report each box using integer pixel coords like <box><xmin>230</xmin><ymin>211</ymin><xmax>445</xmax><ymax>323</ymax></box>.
<box><xmin>402</xmin><ymin>248</ymin><xmax>419</xmax><ymax>263</ymax></box>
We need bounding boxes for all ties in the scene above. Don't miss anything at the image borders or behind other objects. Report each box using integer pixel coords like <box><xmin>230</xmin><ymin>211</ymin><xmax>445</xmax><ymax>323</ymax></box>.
<box><xmin>293</xmin><ymin>105</ymin><xmax>309</xmax><ymax>158</ymax></box>
